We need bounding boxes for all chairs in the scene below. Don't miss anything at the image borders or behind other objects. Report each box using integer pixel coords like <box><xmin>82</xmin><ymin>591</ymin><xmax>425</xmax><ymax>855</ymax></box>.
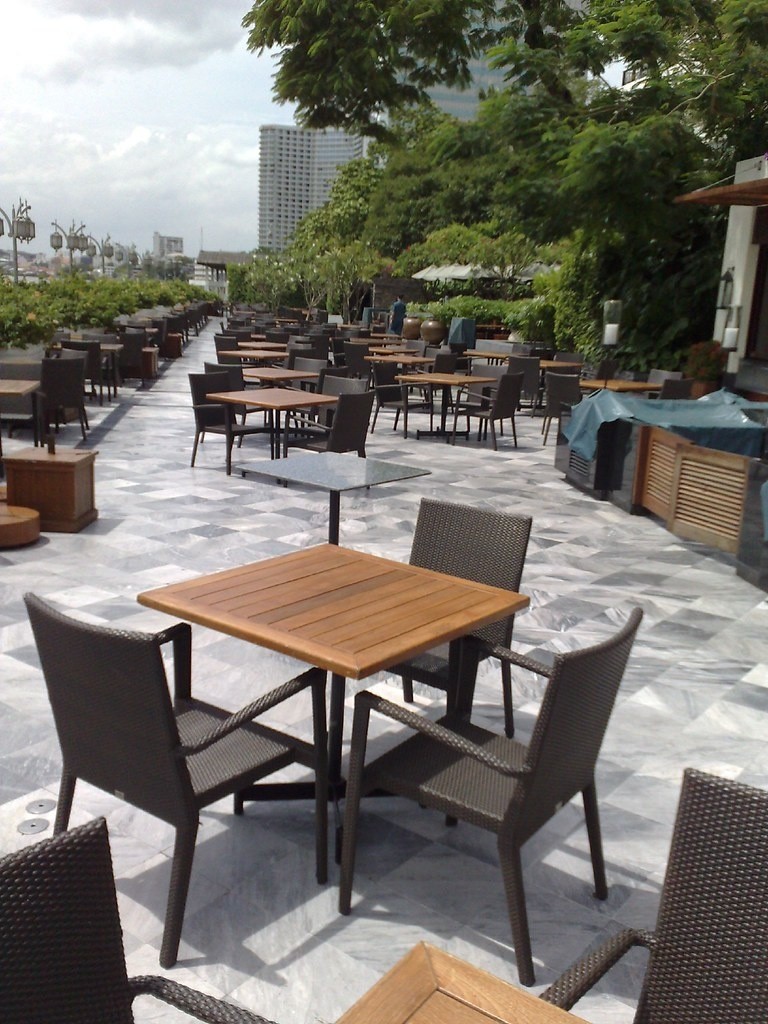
<box><xmin>21</xmin><ymin>592</ymin><xmax>330</xmax><ymax>968</ymax></box>
<box><xmin>188</xmin><ymin>301</ymin><xmax>692</xmax><ymax>476</ymax></box>
<box><xmin>40</xmin><ymin>300</ymin><xmax>210</xmax><ymax>452</ymax></box>
<box><xmin>0</xmin><ymin>816</ymin><xmax>279</xmax><ymax>1024</ymax></box>
<box><xmin>383</xmin><ymin>496</ymin><xmax>532</xmax><ymax>741</ymax></box>
<box><xmin>339</xmin><ymin>606</ymin><xmax>645</xmax><ymax>988</ymax></box>
<box><xmin>537</xmin><ymin>768</ymin><xmax>768</xmax><ymax>1024</ymax></box>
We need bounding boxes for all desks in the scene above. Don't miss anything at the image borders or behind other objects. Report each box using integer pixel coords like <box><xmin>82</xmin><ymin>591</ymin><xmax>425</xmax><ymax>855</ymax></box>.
<box><xmin>242</xmin><ymin>367</ymin><xmax>319</xmax><ymax>428</ymax></box>
<box><xmin>100</xmin><ymin>343</ymin><xmax>124</xmax><ymax>400</ymax></box>
<box><xmin>238</xmin><ymin>342</ymin><xmax>287</xmax><ymax>349</ymax></box>
<box><xmin>463</xmin><ymin>351</ymin><xmax>509</xmax><ymax>366</ymax></box>
<box><xmin>333</xmin><ymin>939</ymin><xmax>594</xmax><ymax>1024</ymax></box>
<box><xmin>580</xmin><ymin>379</ymin><xmax>663</xmax><ymax>392</ymax></box>
<box><xmin>1</xmin><ymin>446</ymin><xmax>99</xmax><ymax>533</ymax></box>
<box><xmin>369</xmin><ymin>347</ymin><xmax>420</xmax><ymax>354</ymax></box>
<box><xmin>370</xmin><ymin>333</ymin><xmax>400</xmax><ymax>337</ymax></box>
<box><xmin>350</xmin><ymin>338</ymin><xmax>401</xmax><ymax>345</ymax></box>
<box><xmin>364</xmin><ymin>356</ymin><xmax>435</xmax><ymax>370</ymax></box>
<box><xmin>218</xmin><ymin>350</ymin><xmax>288</xmax><ymax>367</ymax></box>
<box><xmin>235</xmin><ymin>450</ymin><xmax>430</xmax><ymax>546</ymax></box>
<box><xmin>0</xmin><ymin>380</ymin><xmax>41</xmax><ymax>437</ymax></box>
<box><xmin>540</xmin><ymin>359</ymin><xmax>584</xmax><ymax>386</ymax></box>
<box><xmin>206</xmin><ymin>388</ymin><xmax>341</xmax><ymax>484</ymax></box>
<box><xmin>394</xmin><ymin>373</ymin><xmax>499</xmax><ymax>441</ymax></box>
<box><xmin>137</xmin><ymin>543</ymin><xmax>530</xmax><ymax>866</ymax></box>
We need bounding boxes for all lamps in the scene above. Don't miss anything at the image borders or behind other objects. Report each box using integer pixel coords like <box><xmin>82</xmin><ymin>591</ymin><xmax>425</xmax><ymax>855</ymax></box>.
<box><xmin>717</xmin><ymin>267</ymin><xmax>735</xmax><ymax>309</ymax></box>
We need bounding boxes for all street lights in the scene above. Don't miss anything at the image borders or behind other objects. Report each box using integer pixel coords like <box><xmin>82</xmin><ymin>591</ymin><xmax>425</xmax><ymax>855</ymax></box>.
<box><xmin>0</xmin><ymin>196</ymin><xmax>35</xmax><ymax>286</ymax></box>
<box><xmin>87</xmin><ymin>233</ymin><xmax>113</xmax><ymax>274</ymax></box>
<box><xmin>115</xmin><ymin>242</ymin><xmax>136</xmax><ymax>264</ymax></box>
<box><xmin>50</xmin><ymin>219</ymin><xmax>88</xmax><ymax>274</ymax></box>
